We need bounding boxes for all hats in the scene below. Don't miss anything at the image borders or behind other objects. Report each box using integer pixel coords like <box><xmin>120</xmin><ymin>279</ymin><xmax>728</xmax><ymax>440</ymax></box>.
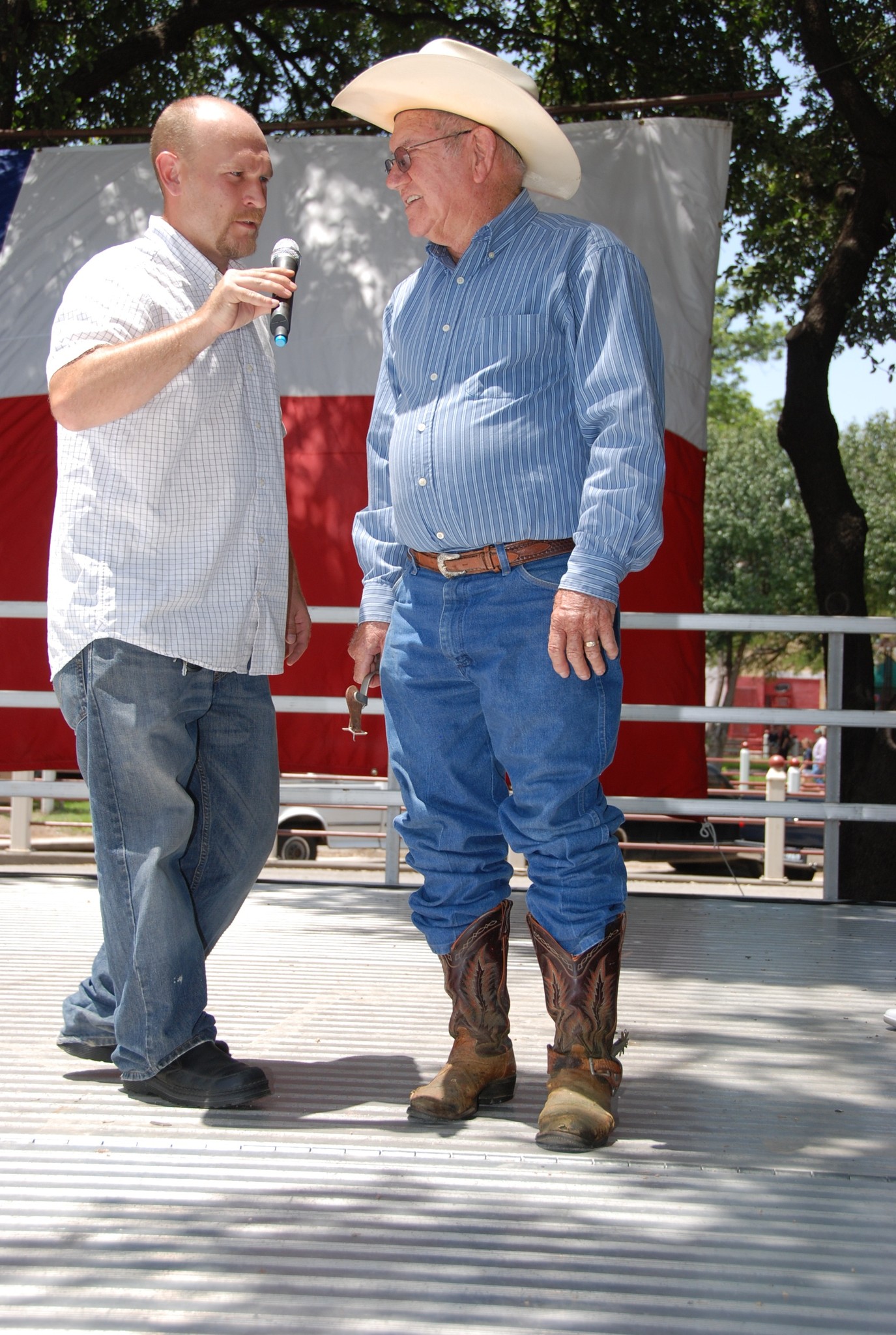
<box><xmin>331</xmin><ymin>38</ymin><xmax>581</xmax><ymax>202</ymax></box>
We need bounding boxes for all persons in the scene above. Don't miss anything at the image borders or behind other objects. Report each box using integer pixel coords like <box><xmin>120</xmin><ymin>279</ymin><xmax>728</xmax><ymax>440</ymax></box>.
<box><xmin>334</xmin><ymin>38</ymin><xmax>667</xmax><ymax>1152</ymax></box>
<box><xmin>870</xmin><ymin>631</ymin><xmax>896</xmax><ymax>753</ymax></box>
<box><xmin>761</xmin><ymin>723</ymin><xmax>829</xmax><ymax>789</ymax></box>
<box><xmin>47</xmin><ymin>95</ymin><xmax>312</xmax><ymax>1108</ymax></box>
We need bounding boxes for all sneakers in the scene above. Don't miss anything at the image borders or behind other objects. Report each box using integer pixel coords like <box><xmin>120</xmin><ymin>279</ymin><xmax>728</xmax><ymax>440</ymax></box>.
<box><xmin>59</xmin><ymin>1024</ymin><xmax>117</xmax><ymax>1063</ymax></box>
<box><xmin>129</xmin><ymin>1039</ymin><xmax>272</xmax><ymax>1111</ymax></box>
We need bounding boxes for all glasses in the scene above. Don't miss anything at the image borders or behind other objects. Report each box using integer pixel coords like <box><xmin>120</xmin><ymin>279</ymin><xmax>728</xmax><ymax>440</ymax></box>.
<box><xmin>384</xmin><ymin>129</ymin><xmax>470</xmax><ymax>175</ymax></box>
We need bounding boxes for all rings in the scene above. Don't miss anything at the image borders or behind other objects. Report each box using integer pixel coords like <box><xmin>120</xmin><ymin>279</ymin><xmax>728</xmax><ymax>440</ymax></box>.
<box><xmin>582</xmin><ymin>640</ymin><xmax>601</xmax><ymax>649</ymax></box>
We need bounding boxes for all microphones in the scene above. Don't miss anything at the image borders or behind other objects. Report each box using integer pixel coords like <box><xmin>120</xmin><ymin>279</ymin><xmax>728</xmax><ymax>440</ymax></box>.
<box><xmin>269</xmin><ymin>238</ymin><xmax>301</xmax><ymax>348</ymax></box>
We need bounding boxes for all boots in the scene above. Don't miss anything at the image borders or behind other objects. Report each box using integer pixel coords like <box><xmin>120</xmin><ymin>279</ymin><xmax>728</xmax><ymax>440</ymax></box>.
<box><xmin>407</xmin><ymin>900</ymin><xmax>517</xmax><ymax>1124</ymax></box>
<box><xmin>525</xmin><ymin>913</ymin><xmax>630</xmax><ymax>1151</ymax></box>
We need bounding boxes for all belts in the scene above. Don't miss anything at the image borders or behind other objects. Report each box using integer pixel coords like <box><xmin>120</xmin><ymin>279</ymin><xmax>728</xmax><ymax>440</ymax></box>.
<box><xmin>404</xmin><ymin>534</ymin><xmax>574</xmax><ymax>580</ymax></box>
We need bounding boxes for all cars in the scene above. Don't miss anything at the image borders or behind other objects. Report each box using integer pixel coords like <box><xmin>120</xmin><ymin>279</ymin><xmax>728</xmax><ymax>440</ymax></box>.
<box><xmin>615</xmin><ymin>762</ymin><xmax>824</xmax><ymax>881</ymax></box>
<box><xmin>266</xmin><ymin>773</ymin><xmax>412</xmax><ymax>862</ymax></box>
